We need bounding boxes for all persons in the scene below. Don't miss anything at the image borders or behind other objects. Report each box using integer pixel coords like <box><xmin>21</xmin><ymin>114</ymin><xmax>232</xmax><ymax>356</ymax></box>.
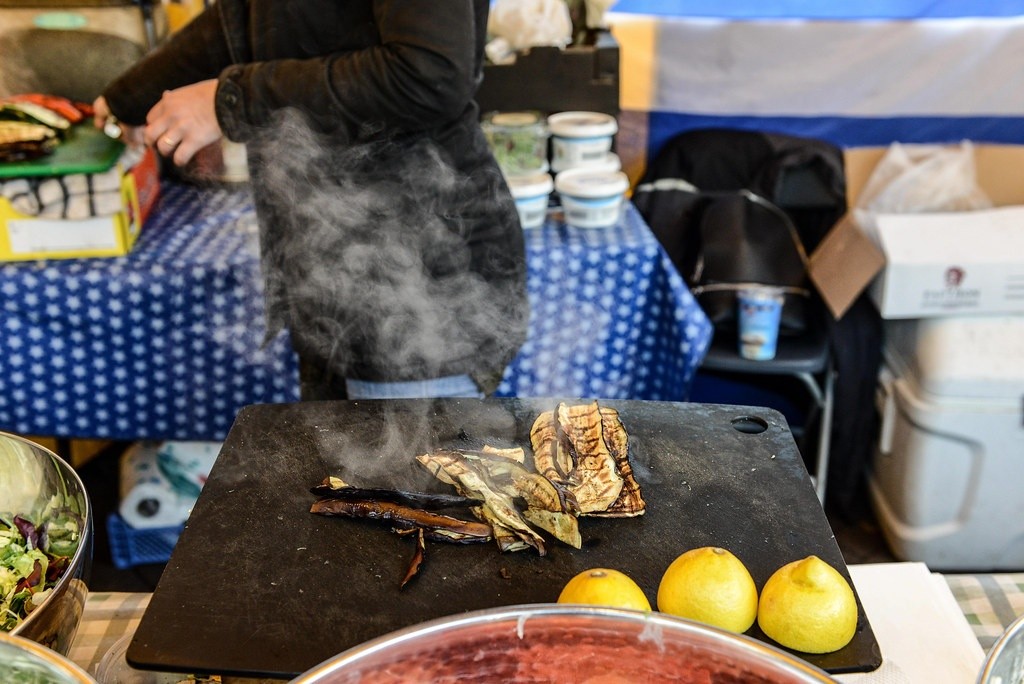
<box><xmin>92</xmin><ymin>1</ymin><xmax>529</xmax><ymax>401</ymax></box>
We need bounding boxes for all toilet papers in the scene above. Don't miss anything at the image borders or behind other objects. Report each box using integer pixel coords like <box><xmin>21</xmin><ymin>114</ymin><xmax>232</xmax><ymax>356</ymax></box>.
<box><xmin>118</xmin><ymin>444</ymin><xmax>175</xmax><ymax>529</ymax></box>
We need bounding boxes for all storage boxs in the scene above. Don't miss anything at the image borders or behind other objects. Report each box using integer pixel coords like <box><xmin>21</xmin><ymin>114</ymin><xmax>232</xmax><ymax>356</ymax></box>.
<box><xmin>0</xmin><ymin>136</ymin><xmax>162</xmax><ymax>261</ymax></box>
<box><xmin>802</xmin><ymin>137</ymin><xmax>1024</xmax><ymax>322</ymax></box>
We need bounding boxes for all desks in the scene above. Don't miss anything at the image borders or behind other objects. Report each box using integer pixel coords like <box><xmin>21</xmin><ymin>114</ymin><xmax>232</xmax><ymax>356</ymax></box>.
<box><xmin>0</xmin><ymin>175</ymin><xmax>710</xmax><ymax>439</ymax></box>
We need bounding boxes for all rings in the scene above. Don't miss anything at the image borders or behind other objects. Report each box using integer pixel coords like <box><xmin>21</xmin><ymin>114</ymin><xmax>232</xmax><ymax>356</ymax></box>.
<box><xmin>162</xmin><ymin>137</ymin><xmax>175</xmax><ymax>147</ymax></box>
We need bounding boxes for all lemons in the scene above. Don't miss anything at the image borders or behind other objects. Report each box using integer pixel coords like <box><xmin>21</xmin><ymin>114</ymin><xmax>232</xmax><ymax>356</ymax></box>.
<box><xmin>757</xmin><ymin>555</ymin><xmax>857</xmax><ymax>653</ymax></box>
<box><xmin>556</xmin><ymin>568</ymin><xmax>652</xmax><ymax>610</ymax></box>
<box><xmin>656</xmin><ymin>546</ymin><xmax>759</xmax><ymax>634</ymax></box>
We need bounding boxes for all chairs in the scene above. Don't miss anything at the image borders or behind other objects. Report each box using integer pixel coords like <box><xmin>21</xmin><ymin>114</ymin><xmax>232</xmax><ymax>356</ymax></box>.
<box><xmin>634</xmin><ymin>127</ymin><xmax>840</xmax><ymax>508</ymax></box>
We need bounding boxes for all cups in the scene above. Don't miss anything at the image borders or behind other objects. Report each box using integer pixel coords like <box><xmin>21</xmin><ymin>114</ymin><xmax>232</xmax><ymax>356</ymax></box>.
<box><xmin>738</xmin><ymin>289</ymin><xmax>786</xmax><ymax>361</ymax></box>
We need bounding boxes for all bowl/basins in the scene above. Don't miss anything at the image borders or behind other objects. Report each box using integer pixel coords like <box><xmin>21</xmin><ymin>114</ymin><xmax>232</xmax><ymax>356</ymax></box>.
<box><xmin>94</xmin><ymin>629</ymin><xmax>289</xmax><ymax>684</ymax></box>
<box><xmin>0</xmin><ymin>630</ymin><xmax>99</xmax><ymax>684</ymax></box>
<box><xmin>0</xmin><ymin>429</ymin><xmax>95</xmax><ymax>660</ymax></box>
<box><xmin>279</xmin><ymin>602</ymin><xmax>840</xmax><ymax>683</ymax></box>
<box><xmin>478</xmin><ymin>108</ymin><xmax>629</xmax><ymax>230</ymax></box>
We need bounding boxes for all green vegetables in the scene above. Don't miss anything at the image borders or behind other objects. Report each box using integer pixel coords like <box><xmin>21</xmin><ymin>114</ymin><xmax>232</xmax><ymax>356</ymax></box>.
<box><xmin>0</xmin><ymin>456</ymin><xmax>79</xmax><ymax>635</ymax></box>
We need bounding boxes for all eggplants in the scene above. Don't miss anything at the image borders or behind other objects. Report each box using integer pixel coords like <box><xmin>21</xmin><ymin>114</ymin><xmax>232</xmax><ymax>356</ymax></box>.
<box><xmin>310</xmin><ymin>400</ymin><xmax>649</xmax><ymax>588</ymax></box>
